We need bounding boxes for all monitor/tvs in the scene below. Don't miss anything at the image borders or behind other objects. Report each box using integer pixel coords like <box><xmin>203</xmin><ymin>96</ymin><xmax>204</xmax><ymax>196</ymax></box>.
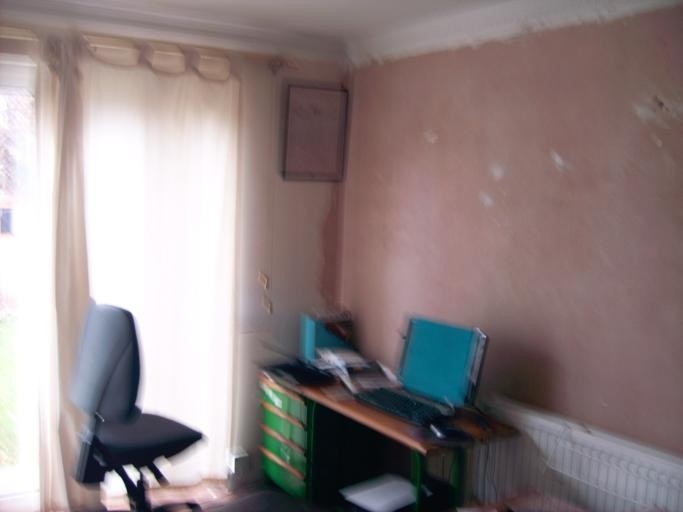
<box><xmin>400</xmin><ymin>318</ymin><xmax>490</xmax><ymax>410</ymax></box>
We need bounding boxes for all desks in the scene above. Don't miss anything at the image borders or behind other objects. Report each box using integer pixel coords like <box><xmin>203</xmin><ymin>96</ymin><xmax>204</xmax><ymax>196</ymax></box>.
<box><xmin>258</xmin><ymin>359</ymin><xmax>523</xmax><ymax>512</ymax></box>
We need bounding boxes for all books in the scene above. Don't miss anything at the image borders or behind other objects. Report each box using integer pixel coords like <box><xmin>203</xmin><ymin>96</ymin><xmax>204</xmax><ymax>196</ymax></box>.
<box><xmin>311</xmin><ymin>346</ymin><xmax>404</xmax><ymax>397</ymax></box>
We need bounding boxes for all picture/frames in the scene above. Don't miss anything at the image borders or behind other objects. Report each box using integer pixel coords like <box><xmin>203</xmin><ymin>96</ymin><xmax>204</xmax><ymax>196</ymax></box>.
<box><xmin>280</xmin><ymin>84</ymin><xmax>350</xmax><ymax>182</ymax></box>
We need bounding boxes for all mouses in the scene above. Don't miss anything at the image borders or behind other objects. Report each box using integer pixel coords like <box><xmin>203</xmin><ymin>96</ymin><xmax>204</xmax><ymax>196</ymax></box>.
<box><xmin>427</xmin><ymin>423</ymin><xmax>450</xmax><ymax>446</ymax></box>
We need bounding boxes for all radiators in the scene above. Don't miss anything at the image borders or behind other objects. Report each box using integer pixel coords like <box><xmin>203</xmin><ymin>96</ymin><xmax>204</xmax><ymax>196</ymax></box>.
<box><xmin>464</xmin><ymin>387</ymin><xmax>683</xmax><ymax>511</ymax></box>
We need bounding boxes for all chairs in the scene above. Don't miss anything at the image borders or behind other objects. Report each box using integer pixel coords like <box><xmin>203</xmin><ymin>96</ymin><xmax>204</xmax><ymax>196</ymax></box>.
<box><xmin>71</xmin><ymin>304</ymin><xmax>203</xmax><ymax>512</ymax></box>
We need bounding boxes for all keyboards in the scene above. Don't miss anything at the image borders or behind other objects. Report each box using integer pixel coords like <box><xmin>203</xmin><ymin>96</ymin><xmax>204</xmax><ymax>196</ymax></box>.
<box><xmin>355</xmin><ymin>384</ymin><xmax>448</xmax><ymax>427</ymax></box>
